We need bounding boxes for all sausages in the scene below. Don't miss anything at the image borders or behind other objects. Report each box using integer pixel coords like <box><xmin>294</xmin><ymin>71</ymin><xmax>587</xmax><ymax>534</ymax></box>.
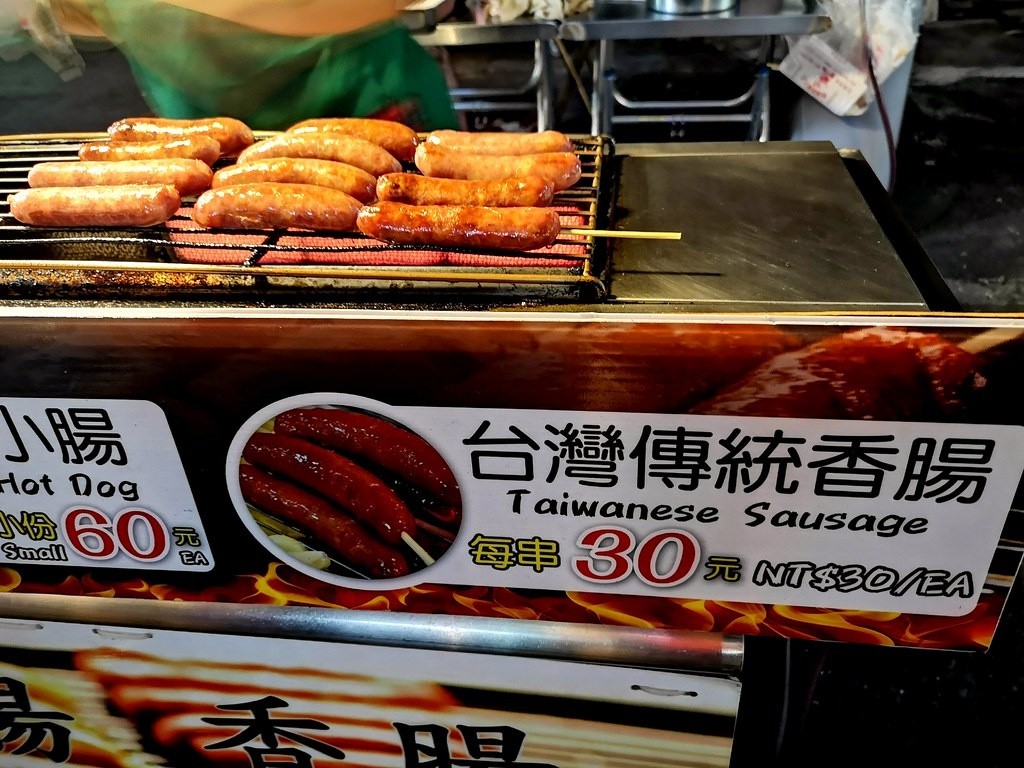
<box><xmin>239</xmin><ymin>409</ymin><xmax>454</xmax><ymax>579</ymax></box>
<box><xmin>8</xmin><ymin>116</ymin><xmax>255</xmax><ymax>229</ymax></box>
<box><xmin>357</xmin><ymin>129</ymin><xmax>582</xmax><ymax>251</ymax></box>
<box><xmin>191</xmin><ymin>117</ymin><xmax>422</xmax><ymax>233</ymax></box>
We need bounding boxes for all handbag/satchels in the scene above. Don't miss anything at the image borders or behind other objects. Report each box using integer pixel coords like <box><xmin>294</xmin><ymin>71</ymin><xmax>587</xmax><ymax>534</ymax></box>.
<box><xmin>778</xmin><ymin>0</ymin><xmax>919</xmax><ymax>117</ymax></box>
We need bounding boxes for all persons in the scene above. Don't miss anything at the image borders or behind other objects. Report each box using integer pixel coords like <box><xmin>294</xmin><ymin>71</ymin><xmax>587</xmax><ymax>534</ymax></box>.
<box><xmin>0</xmin><ymin>1</ymin><xmax>147</xmax><ymax>133</ymax></box>
<box><xmin>96</xmin><ymin>1</ymin><xmax>459</xmax><ymax>135</ymax></box>
